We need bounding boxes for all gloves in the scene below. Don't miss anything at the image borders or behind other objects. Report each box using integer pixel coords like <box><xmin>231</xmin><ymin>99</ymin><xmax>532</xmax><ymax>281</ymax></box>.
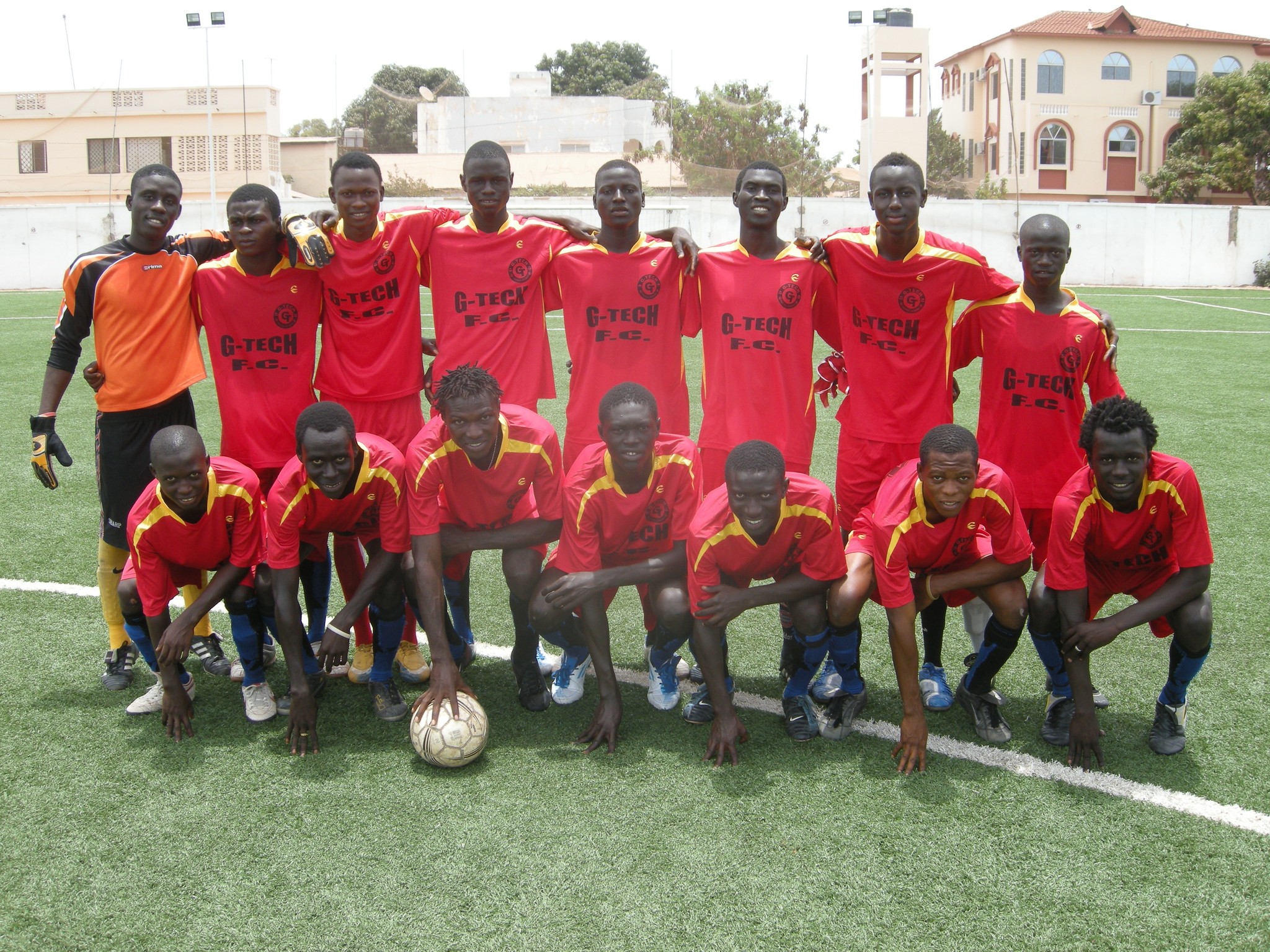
<box><xmin>283</xmin><ymin>215</ymin><xmax>334</xmax><ymax>269</ymax></box>
<box><xmin>30</xmin><ymin>412</ymin><xmax>73</xmax><ymax>492</ymax></box>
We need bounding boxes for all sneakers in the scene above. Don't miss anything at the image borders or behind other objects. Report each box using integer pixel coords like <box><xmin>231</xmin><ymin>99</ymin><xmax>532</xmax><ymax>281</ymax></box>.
<box><xmin>277</xmin><ymin>669</ymin><xmax>327</xmax><ymax>713</ymax></box>
<box><xmin>963</xmin><ymin>651</ymin><xmax>995</xmax><ymax>685</ymax></box>
<box><xmin>780</xmin><ymin>685</ymin><xmax>818</xmax><ymax>743</ymax></box>
<box><xmin>955</xmin><ymin>672</ymin><xmax>1012</xmax><ymax>743</ymax></box>
<box><xmin>307</xmin><ymin>631</ymin><xmax>349</xmax><ymax>678</ymax></box>
<box><xmin>690</xmin><ymin>635</ymin><xmax>728</xmax><ymax>681</ymax></box>
<box><xmin>646</xmin><ymin>645</ymin><xmax>680</xmax><ymax>711</ymax></box>
<box><xmin>779</xmin><ymin>655</ymin><xmax>816</xmax><ymax>691</ymax></box>
<box><xmin>394</xmin><ymin>640</ymin><xmax>430</xmax><ymax>684</ymax></box>
<box><xmin>511</xmin><ymin>644</ymin><xmax>551</xmax><ymax>711</ymax></box>
<box><xmin>535</xmin><ymin>640</ymin><xmax>553</xmax><ymax>675</ymax></box>
<box><xmin>643</xmin><ymin>634</ymin><xmax>689</xmax><ymax>677</ymax></box>
<box><xmin>683</xmin><ymin>677</ymin><xmax>735</xmax><ymax>724</ymax></box>
<box><xmin>818</xmin><ymin>676</ymin><xmax>867</xmax><ymax>740</ymax></box>
<box><xmin>1044</xmin><ymin>672</ymin><xmax>1110</xmax><ymax>708</ymax></box>
<box><xmin>101</xmin><ymin>630</ymin><xmax>279</xmax><ymax>724</ymax></box>
<box><xmin>813</xmin><ymin>660</ymin><xmax>843</xmax><ymax>701</ymax></box>
<box><xmin>550</xmin><ymin>653</ymin><xmax>564</xmax><ymax>672</ymax></box>
<box><xmin>918</xmin><ymin>661</ymin><xmax>953</xmax><ymax>710</ymax></box>
<box><xmin>1041</xmin><ymin>692</ymin><xmax>1076</xmax><ymax>745</ymax></box>
<box><xmin>552</xmin><ymin>648</ymin><xmax>592</xmax><ymax>704</ymax></box>
<box><xmin>430</xmin><ymin>641</ymin><xmax>475</xmax><ymax>673</ymax></box>
<box><xmin>366</xmin><ymin>676</ymin><xmax>408</xmax><ymax>721</ymax></box>
<box><xmin>347</xmin><ymin>642</ymin><xmax>373</xmax><ymax>683</ymax></box>
<box><xmin>1150</xmin><ymin>687</ymin><xmax>1187</xmax><ymax>755</ymax></box>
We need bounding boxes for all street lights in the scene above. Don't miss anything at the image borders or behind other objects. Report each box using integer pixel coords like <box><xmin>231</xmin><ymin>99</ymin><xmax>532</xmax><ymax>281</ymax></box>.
<box><xmin>846</xmin><ymin>10</ymin><xmax>887</xmax><ymax>225</ymax></box>
<box><xmin>186</xmin><ymin>11</ymin><xmax>225</xmax><ymax>231</ymax></box>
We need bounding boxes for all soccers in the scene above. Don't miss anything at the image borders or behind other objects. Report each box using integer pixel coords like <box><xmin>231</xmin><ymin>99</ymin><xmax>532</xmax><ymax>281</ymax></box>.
<box><xmin>410</xmin><ymin>691</ymin><xmax>489</xmax><ymax>767</ymax></box>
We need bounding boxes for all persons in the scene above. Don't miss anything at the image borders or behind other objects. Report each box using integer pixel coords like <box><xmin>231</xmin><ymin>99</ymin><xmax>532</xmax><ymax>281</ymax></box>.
<box><xmin>526</xmin><ymin>384</ymin><xmax>702</xmax><ymax>755</ymax></box>
<box><xmin>820</xmin><ymin>423</ymin><xmax>1035</xmax><ymax>778</ymax></box>
<box><xmin>265</xmin><ymin>400</ymin><xmax>410</xmax><ymax>756</ymax></box>
<box><xmin>688</xmin><ymin>441</ymin><xmax>849</xmax><ymax>766</ymax></box>
<box><xmin>84</xmin><ymin>142</ymin><xmax>1130</xmax><ymax>715</ymax></box>
<box><xmin>403</xmin><ymin>361</ymin><xmax>566</xmax><ymax>729</ymax></box>
<box><xmin>114</xmin><ymin>424</ymin><xmax>278</xmax><ymax>742</ymax></box>
<box><xmin>1026</xmin><ymin>393</ymin><xmax>1214</xmax><ymax>772</ymax></box>
<box><xmin>28</xmin><ymin>163</ymin><xmax>333</xmax><ymax>693</ymax></box>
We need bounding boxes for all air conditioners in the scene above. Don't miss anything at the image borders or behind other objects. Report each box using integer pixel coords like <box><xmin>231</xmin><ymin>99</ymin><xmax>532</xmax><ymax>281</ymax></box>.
<box><xmin>1140</xmin><ymin>89</ymin><xmax>1162</xmax><ymax>106</ymax></box>
<box><xmin>974</xmin><ymin>142</ymin><xmax>986</xmax><ymax>155</ymax></box>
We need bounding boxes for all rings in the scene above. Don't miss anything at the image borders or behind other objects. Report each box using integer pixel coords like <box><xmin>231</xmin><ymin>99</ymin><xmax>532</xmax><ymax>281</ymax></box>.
<box><xmin>1074</xmin><ymin>645</ymin><xmax>1084</xmax><ymax>652</ymax></box>
<box><xmin>300</xmin><ymin>733</ymin><xmax>308</xmax><ymax>736</ymax></box>
<box><xmin>1109</xmin><ymin>344</ymin><xmax>1116</xmax><ymax>349</ymax></box>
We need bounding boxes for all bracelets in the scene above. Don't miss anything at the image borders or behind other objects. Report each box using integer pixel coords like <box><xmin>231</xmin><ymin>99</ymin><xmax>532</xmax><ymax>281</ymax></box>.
<box><xmin>326</xmin><ymin>624</ymin><xmax>351</xmax><ymax>639</ymax></box>
<box><xmin>926</xmin><ymin>573</ymin><xmax>940</xmax><ymax>600</ymax></box>
